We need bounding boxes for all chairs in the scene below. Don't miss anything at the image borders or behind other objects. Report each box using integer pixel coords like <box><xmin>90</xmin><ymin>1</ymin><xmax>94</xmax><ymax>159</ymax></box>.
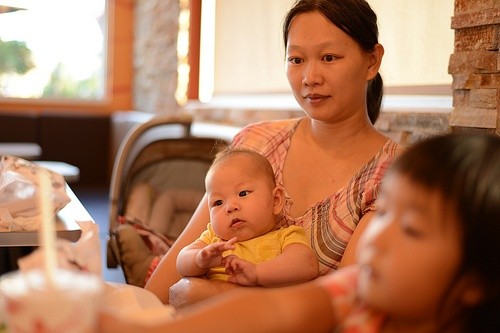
<box><xmin>110</xmin><ymin>113</ymin><xmax>195</xmax><ymax>263</ymax></box>
<box><xmin>111</xmin><ymin>134</ymin><xmax>230</xmax><ymax>288</ymax></box>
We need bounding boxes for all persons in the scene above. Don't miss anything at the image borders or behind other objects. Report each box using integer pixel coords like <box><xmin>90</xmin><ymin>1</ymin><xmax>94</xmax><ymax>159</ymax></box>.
<box><xmin>175</xmin><ymin>145</ymin><xmax>319</xmax><ymax>288</ymax></box>
<box><xmin>143</xmin><ymin>0</ymin><xmax>409</xmax><ymax>318</ymax></box>
<box><xmin>94</xmin><ymin>131</ymin><xmax>500</xmax><ymax>333</ymax></box>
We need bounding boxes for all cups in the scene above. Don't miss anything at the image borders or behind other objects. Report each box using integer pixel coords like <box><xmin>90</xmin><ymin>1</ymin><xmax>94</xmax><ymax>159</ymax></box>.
<box><xmin>0</xmin><ymin>267</ymin><xmax>108</xmax><ymax>333</ymax></box>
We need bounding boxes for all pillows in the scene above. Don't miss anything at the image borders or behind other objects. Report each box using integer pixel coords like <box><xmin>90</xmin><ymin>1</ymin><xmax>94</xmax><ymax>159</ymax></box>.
<box><xmin>116</xmin><ymin>212</ymin><xmax>177</xmax><ymax>259</ymax></box>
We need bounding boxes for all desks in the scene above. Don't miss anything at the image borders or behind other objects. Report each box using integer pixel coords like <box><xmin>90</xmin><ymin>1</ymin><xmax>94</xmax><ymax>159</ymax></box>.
<box><xmin>0</xmin><ymin>143</ymin><xmax>95</xmax><ymax>275</ymax></box>
